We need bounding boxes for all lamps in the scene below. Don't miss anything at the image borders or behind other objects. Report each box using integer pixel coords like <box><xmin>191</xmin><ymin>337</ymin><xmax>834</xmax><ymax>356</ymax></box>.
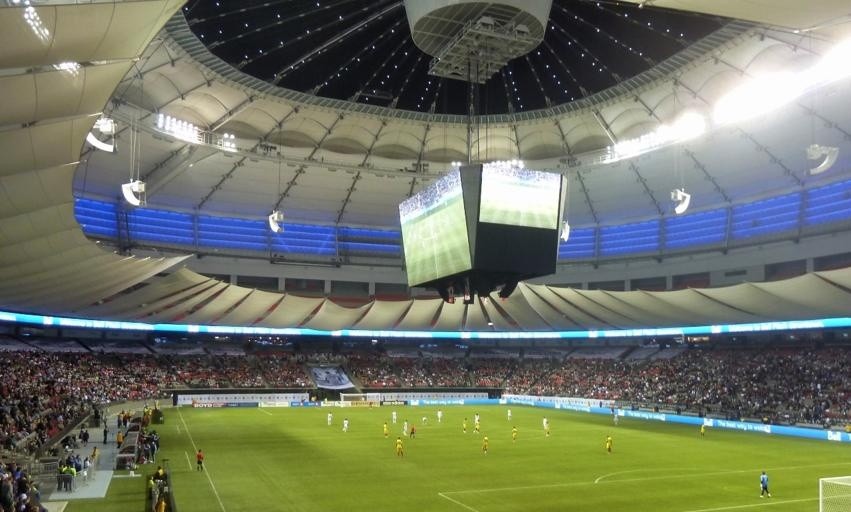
<box><xmin>121</xmin><ymin>84</ymin><xmax>151</xmax><ymax>210</ymax></box>
<box><xmin>805</xmin><ymin>29</ymin><xmax>841</xmax><ymax>177</ymax></box>
<box><xmin>670</xmin><ymin>78</ymin><xmax>694</xmax><ymax>214</ymax></box>
<box><xmin>266</xmin><ymin>119</ymin><xmax>287</xmax><ymax>235</ymax></box>
<box><xmin>86</xmin><ymin>69</ymin><xmax>126</xmax><ymax>156</ymax></box>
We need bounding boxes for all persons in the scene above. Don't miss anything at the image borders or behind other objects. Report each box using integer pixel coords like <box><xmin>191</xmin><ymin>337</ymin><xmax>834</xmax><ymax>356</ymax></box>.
<box><xmin>399</xmin><ymin>164</ymin><xmax>562</xmax><ymax>220</ymax></box>
<box><xmin>188</xmin><ymin>343</ymin><xmax>851</xmax><ymax>434</ymax></box>
<box><xmin>759</xmin><ymin>471</ymin><xmax>773</xmax><ymax>498</ymax></box>
<box><xmin>505</xmin><ymin>407</ymin><xmax>618</xmax><ymax>453</ymax></box>
<box><xmin>381</xmin><ymin>407</ymin><xmax>489</xmax><ymax>457</ymax></box>
<box><xmin>700</xmin><ymin>424</ymin><xmax>705</xmax><ymax>437</ymax></box>
<box><xmin>1</xmin><ymin>343</ymin><xmax>204</xmax><ymax>511</ymax></box>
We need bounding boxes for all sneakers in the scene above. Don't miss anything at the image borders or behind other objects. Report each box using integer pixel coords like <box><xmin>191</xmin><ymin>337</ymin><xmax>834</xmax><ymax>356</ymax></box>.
<box><xmin>760</xmin><ymin>496</ymin><xmax>763</xmax><ymax>498</ymax></box>
<box><xmin>768</xmin><ymin>494</ymin><xmax>771</xmax><ymax>498</ymax></box>
